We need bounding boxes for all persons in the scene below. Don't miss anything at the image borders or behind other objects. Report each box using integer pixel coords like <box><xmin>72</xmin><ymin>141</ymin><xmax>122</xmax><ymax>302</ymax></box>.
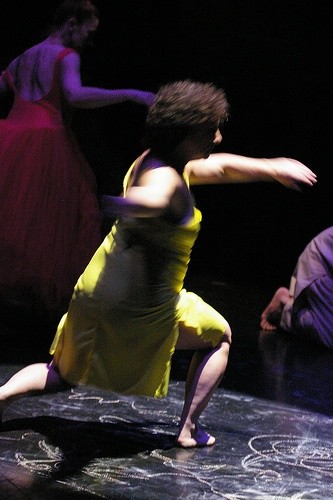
<box><xmin>0</xmin><ymin>0</ymin><xmax>157</xmax><ymax>306</ymax></box>
<box><xmin>259</xmin><ymin>227</ymin><xmax>333</xmax><ymax>348</ymax></box>
<box><xmin>0</xmin><ymin>82</ymin><xmax>317</xmax><ymax>446</ymax></box>
